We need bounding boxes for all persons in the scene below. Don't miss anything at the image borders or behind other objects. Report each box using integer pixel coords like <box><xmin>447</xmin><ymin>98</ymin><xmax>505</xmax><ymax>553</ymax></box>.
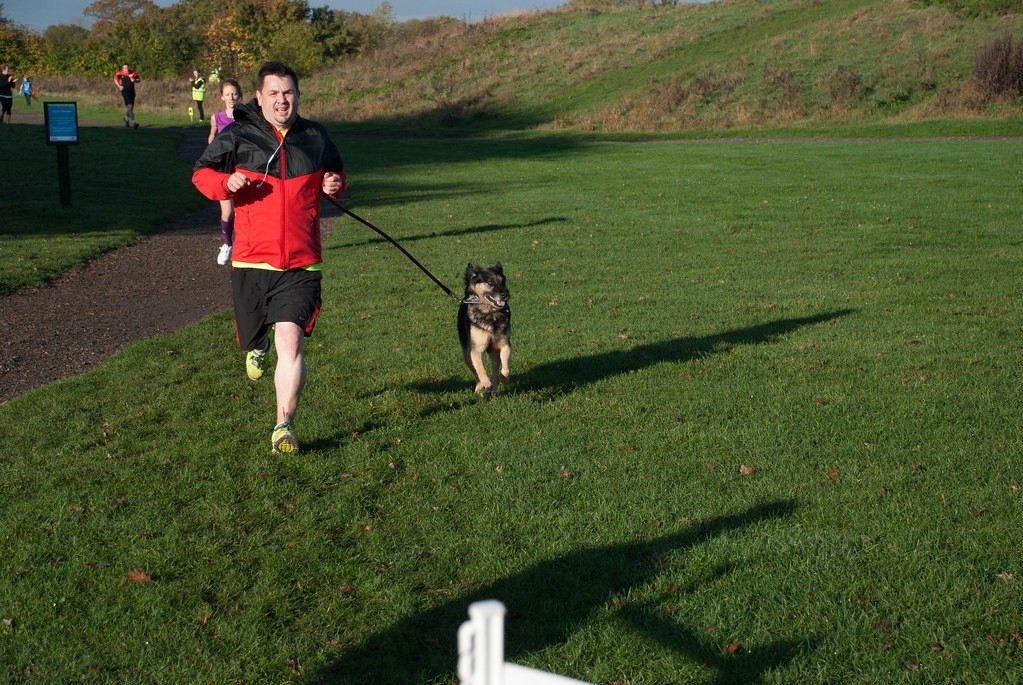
<box><xmin>0</xmin><ymin>64</ymin><xmax>18</xmax><ymax>124</ymax></box>
<box><xmin>19</xmin><ymin>76</ymin><xmax>34</xmax><ymax>106</ymax></box>
<box><xmin>189</xmin><ymin>62</ymin><xmax>346</xmax><ymax>455</ymax></box>
<box><xmin>114</xmin><ymin>63</ymin><xmax>140</xmax><ymax>129</ymax></box>
<box><xmin>207</xmin><ymin>79</ymin><xmax>244</xmax><ymax>266</ymax></box>
<box><xmin>189</xmin><ymin>69</ymin><xmax>205</xmax><ymax>122</ymax></box>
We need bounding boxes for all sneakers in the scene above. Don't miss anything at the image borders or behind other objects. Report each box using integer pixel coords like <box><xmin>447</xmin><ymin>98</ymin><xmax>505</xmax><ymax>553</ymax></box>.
<box><xmin>123</xmin><ymin>117</ymin><xmax>130</xmax><ymax>128</ymax></box>
<box><xmin>270</xmin><ymin>423</ymin><xmax>299</xmax><ymax>458</ymax></box>
<box><xmin>217</xmin><ymin>244</ymin><xmax>234</xmax><ymax>265</ymax></box>
<box><xmin>247</xmin><ymin>339</ymin><xmax>270</xmax><ymax>380</ymax></box>
<box><xmin>134</xmin><ymin>124</ymin><xmax>139</xmax><ymax>129</ymax></box>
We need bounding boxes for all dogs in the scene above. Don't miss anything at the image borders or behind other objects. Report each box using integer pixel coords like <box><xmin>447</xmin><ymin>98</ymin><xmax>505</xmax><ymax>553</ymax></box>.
<box><xmin>457</xmin><ymin>261</ymin><xmax>512</xmax><ymax>397</ymax></box>
